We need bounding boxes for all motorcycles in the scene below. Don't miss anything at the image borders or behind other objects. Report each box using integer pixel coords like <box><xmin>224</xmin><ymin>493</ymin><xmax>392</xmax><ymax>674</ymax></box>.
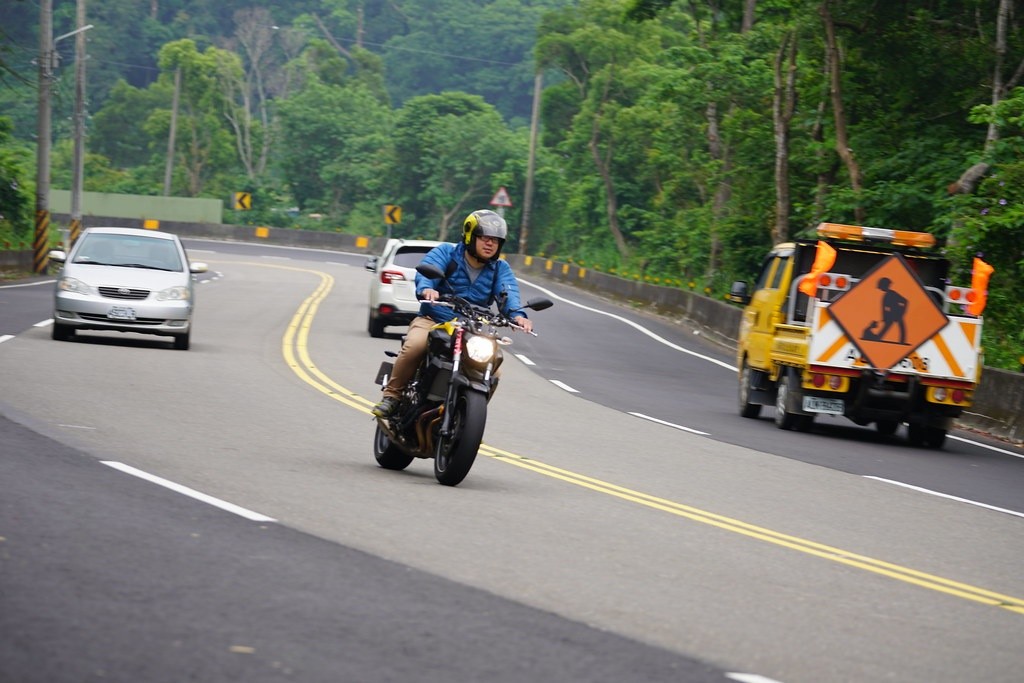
<box><xmin>371</xmin><ymin>263</ymin><xmax>554</xmax><ymax>487</ymax></box>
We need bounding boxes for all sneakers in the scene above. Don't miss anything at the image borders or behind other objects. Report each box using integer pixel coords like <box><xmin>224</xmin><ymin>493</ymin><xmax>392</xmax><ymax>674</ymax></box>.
<box><xmin>371</xmin><ymin>397</ymin><xmax>402</xmax><ymax>418</ymax></box>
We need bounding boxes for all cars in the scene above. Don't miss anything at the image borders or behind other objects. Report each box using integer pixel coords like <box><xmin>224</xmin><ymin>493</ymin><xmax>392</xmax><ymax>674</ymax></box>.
<box><xmin>364</xmin><ymin>238</ymin><xmax>458</xmax><ymax>336</ymax></box>
<box><xmin>46</xmin><ymin>226</ymin><xmax>208</xmax><ymax>352</ymax></box>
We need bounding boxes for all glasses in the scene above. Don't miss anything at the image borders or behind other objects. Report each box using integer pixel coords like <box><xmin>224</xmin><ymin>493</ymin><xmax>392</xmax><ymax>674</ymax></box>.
<box><xmin>478</xmin><ymin>234</ymin><xmax>500</xmax><ymax>244</ymax></box>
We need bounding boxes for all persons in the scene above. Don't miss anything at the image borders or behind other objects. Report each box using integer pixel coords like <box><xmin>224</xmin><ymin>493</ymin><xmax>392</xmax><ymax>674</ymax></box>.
<box><xmin>372</xmin><ymin>210</ymin><xmax>533</xmax><ymax>419</ymax></box>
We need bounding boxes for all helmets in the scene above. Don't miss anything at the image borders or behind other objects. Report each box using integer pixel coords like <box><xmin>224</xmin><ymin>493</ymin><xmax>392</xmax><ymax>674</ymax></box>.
<box><xmin>462</xmin><ymin>210</ymin><xmax>508</xmax><ymax>248</ymax></box>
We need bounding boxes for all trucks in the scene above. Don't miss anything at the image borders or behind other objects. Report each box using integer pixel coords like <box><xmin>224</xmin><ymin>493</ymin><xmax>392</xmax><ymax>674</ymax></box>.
<box><xmin>729</xmin><ymin>237</ymin><xmax>992</xmax><ymax>449</ymax></box>
